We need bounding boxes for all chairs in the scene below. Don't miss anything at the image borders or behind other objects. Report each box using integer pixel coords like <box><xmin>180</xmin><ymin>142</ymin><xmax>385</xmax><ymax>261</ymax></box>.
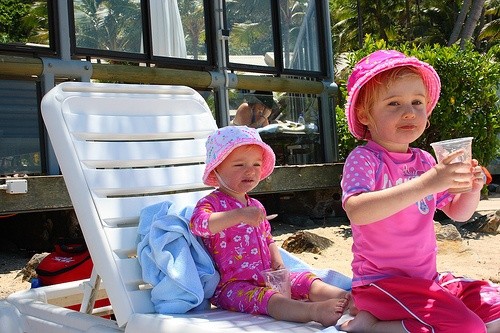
<box><xmin>0</xmin><ymin>85</ymin><xmax>342</xmax><ymax>333</ymax></box>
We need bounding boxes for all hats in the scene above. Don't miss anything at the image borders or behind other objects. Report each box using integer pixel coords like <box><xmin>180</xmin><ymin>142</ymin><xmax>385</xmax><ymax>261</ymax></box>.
<box><xmin>244</xmin><ymin>89</ymin><xmax>278</xmax><ymax>113</ymax></box>
<box><xmin>345</xmin><ymin>50</ymin><xmax>441</xmax><ymax>140</ymax></box>
<box><xmin>201</xmin><ymin>124</ymin><xmax>276</xmax><ymax>186</ymax></box>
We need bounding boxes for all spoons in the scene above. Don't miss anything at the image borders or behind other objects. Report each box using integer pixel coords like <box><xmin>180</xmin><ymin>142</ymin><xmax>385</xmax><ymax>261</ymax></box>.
<box><xmin>238</xmin><ymin>214</ymin><xmax>278</xmax><ymax>227</ymax></box>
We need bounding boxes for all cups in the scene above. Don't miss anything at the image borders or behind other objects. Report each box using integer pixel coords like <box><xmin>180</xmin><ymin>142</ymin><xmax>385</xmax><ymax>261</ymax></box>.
<box><xmin>261</xmin><ymin>267</ymin><xmax>291</xmax><ymax>299</ymax></box>
<box><xmin>430</xmin><ymin>137</ymin><xmax>474</xmax><ymax>193</ymax></box>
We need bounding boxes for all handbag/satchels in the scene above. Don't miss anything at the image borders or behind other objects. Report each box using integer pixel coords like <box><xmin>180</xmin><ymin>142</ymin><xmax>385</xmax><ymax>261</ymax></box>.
<box><xmin>36</xmin><ymin>243</ymin><xmax>111</xmax><ymax>321</ymax></box>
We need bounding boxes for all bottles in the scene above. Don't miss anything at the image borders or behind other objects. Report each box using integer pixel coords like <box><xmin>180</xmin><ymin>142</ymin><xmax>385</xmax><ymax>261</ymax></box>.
<box><xmin>298</xmin><ymin>114</ymin><xmax>305</xmax><ymax>125</ymax></box>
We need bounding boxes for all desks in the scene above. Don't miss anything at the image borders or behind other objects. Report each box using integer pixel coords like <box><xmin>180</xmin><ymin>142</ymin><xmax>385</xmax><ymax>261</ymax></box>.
<box><xmin>258</xmin><ymin>131</ymin><xmax>322</xmax><ymax>166</ymax></box>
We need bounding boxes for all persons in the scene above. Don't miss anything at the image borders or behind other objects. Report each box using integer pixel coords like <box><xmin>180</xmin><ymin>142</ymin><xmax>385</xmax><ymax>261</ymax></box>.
<box><xmin>191</xmin><ymin>126</ymin><xmax>352</xmax><ymax>328</ymax></box>
<box><xmin>229</xmin><ymin>90</ymin><xmax>278</xmax><ymax>130</ymax></box>
<box><xmin>338</xmin><ymin>50</ymin><xmax>500</xmax><ymax>333</ymax></box>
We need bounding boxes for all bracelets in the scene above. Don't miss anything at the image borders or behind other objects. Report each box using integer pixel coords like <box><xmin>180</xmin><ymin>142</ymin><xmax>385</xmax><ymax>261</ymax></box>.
<box><xmin>261</xmin><ymin>115</ymin><xmax>266</xmax><ymax>120</ymax></box>
